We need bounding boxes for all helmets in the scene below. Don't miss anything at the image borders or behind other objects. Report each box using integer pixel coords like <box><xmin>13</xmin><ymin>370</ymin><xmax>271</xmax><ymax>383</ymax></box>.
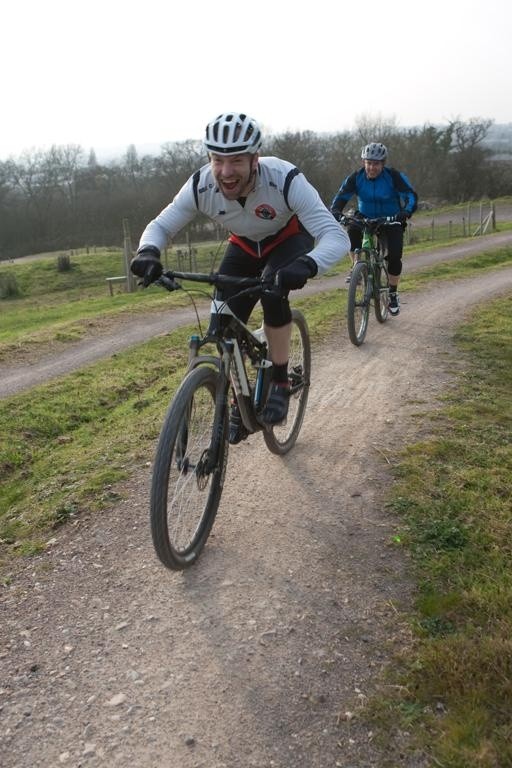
<box><xmin>204</xmin><ymin>111</ymin><xmax>263</xmax><ymax>156</ymax></box>
<box><xmin>360</xmin><ymin>142</ymin><xmax>388</xmax><ymax>160</ymax></box>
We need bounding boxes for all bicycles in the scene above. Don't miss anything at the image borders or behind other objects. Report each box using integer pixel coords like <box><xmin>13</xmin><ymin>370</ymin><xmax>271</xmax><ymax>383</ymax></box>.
<box><xmin>338</xmin><ymin>214</ymin><xmax>401</xmax><ymax>346</ymax></box>
<box><xmin>136</xmin><ymin>268</ymin><xmax>311</xmax><ymax>573</ymax></box>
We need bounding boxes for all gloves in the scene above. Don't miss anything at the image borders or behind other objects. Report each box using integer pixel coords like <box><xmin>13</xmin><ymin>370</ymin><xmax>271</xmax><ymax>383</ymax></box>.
<box><xmin>130</xmin><ymin>244</ymin><xmax>164</xmax><ymax>289</ymax></box>
<box><xmin>331</xmin><ymin>208</ymin><xmax>344</xmax><ymax>222</ymax></box>
<box><xmin>275</xmin><ymin>254</ymin><xmax>318</xmax><ymax>291</ymax></box>
<box><xmin>395</xmin><ymin>211</ymin><xmax>406</xmax><ymax>225</ymax></box>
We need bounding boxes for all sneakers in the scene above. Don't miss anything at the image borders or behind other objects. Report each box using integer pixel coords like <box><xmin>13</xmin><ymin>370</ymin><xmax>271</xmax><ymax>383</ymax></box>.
<box><xmin>346</xmin><ymin>273</ymin><xmax>362</xmax><ymax>285</ymax></box>
<box><xmin>262</xmin><ymin>380</ymin><xmax>291</xmax><ymax>424</ymax></box>
<box><xmin>228</xmin><ymin>392</ymin><xmax>250</xmax><ymax>444</ymax></box>
<box><xmin>387</xmin><ymin>290</ymin><xmax>400</xmax><ymax>317</ymax></box>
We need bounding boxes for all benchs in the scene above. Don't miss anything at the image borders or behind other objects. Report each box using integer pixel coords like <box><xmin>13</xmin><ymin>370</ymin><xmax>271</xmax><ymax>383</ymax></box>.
<box><xmin>106</xmin><ymin>276</ymin><xmax>140</xmax><ymax>296</ymax></box>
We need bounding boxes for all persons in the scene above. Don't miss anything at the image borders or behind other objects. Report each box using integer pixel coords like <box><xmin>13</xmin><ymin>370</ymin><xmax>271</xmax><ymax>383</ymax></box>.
<box><xmin>330</xmin><ymin>142</ymin><xmax>418</xmax><ymax>316</ymax></box>
<box><xmin>131</xmin><ymin>111</ymin><xmax>352</xmax><ymax>446</ymax></box>
